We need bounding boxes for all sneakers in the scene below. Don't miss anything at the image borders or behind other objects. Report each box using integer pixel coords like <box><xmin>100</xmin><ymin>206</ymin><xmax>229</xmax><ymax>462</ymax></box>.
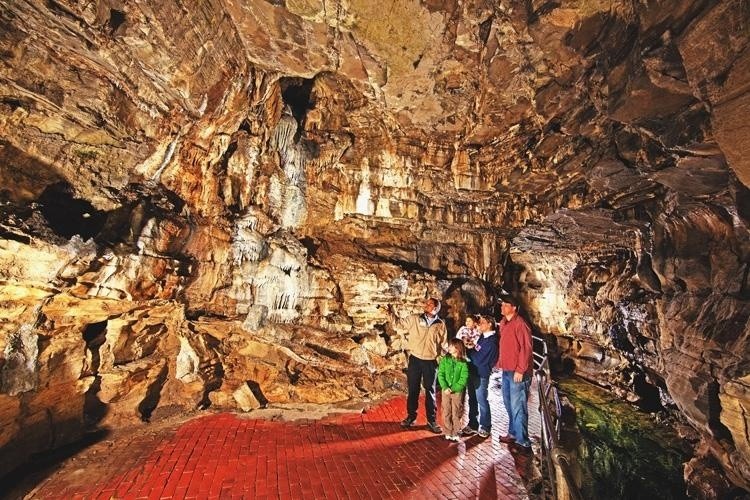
<box><xmin>462</xmin><ymin>426</ymin><xmax>477</xmax><ymax>435</ymax></box>
<box><xmin>499</xmin><ymin>435</ymin><xmax>515</xmax><ymax>443</ymax></box>
<box><xmin>510</xmin><ymin>445</ymin><xmax>530</xmax><ymax>452</ymax></box>
<box><xmin>445</xmin><ymin>435</ymin><xmax>459</xmax><ymax>441</ymax></box>
<box><xmin>479</xmin><ymin>430</ymin><xmax>489</xmax><ymax>437</ymax></box>
<box><xmin>400</xmin><ymin>416</ymin><xmax>416</xmax><ymax>427</ymax></box>
<box><xmin>427</xmin><ymin>422</ymin><xmax>442</xmax><ymax>433</ymax></box>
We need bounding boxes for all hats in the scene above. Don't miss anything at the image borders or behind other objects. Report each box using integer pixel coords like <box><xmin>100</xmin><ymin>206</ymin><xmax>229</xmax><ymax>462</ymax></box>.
<box><xmin>497</xmin><ymin>295</ymin><xmax>518</xmax><ymax>306</ymax></box>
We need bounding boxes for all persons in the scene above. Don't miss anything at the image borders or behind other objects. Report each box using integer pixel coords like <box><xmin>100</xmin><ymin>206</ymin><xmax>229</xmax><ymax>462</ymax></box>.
<box><xmin>380</xmin><ymin>294</ymin><xmax>535</xmax><ymax>454</ymax></box>
